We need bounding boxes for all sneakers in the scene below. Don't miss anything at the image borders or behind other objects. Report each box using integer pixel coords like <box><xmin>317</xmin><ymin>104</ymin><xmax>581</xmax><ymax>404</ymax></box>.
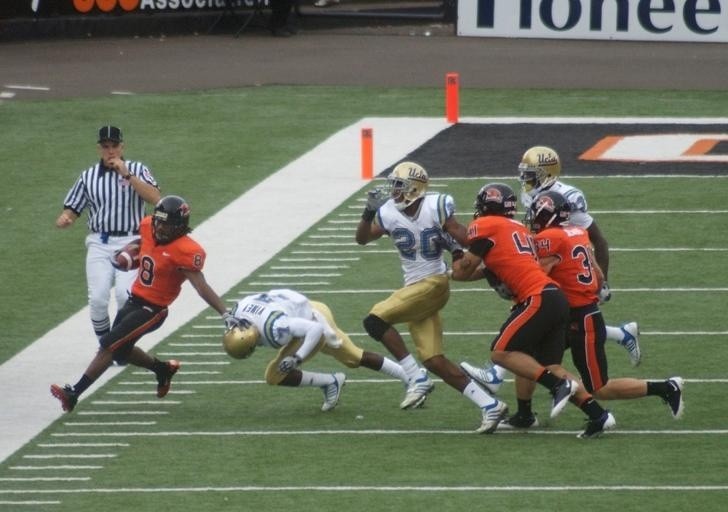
<box><xmin>619</xmin><ymin>321</ymin><xmax>640</xmax><ymax>369</ymax></box>
<box><xmin>399</xmin><ymin>361</ymin><xmax>685</xmax><ymax>438</ymax></box>
<box><xmin>50</xmin><ymin>383</ymin><xmax>77</xmax><ymax>412</ymax></box>
<box><xmin>320</xmin><ymin>373</ymin><xmax>346</xmax><ymax>412</ymax></box>
<box><xmin>157</xmin><ymin>360</ymin><xmax>179</xmax><ymax>399</ymax></box>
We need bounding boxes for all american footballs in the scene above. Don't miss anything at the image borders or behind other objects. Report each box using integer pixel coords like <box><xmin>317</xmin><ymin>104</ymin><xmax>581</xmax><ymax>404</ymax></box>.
<box><xmin>116</xmin><ymin>244</ymin><xmax>140</xmax><ymax>270</ymax></box>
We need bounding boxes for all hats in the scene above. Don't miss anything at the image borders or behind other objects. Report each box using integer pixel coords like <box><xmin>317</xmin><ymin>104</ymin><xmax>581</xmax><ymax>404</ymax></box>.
<box><xmin>97</xmin><ymin>126</ymin><xmax>122</xmax><ymax>143</ymax></box>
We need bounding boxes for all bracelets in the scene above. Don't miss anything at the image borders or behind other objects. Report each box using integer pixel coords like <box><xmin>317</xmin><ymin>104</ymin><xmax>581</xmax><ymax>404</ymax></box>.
<box><xmin>292</xmin><ymin>354</ymin><xmax>303</xmax><ymax>366</ymax></box>
<box><xmin>451</xmin><ymin>249</ymin><xmax>464</xmax><ymax>262</ymax></box>
<box><xmin>361</xmin><ymin>208</ymin><xmax>377</xmax><ymax>222</ymax></box>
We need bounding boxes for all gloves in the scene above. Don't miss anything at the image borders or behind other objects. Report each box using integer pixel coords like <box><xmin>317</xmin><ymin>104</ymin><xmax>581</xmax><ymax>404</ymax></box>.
<box><xmin>598</xmin><ymin>281</ymin><xmax>610</xmax><ymax>302</ymax></box>
<box><xmin>110</xmin><ymin>249</ymin><xmax>128</xmax><ymax>272</ymax></box>
<box><xmin>432</xmin><ymin>229</ymin><xmax>462</xmax><ymax>252</ymax></box>
<box><xmin>365</xmin><ymin>190</ymin><xmax>389</xmax><ymax>211</ymax></box>
<box><xmin>222</xmin><ymin>312</ymin><xmax>251</xmax><ymax>332</ymax></box>
<box><xmin>275</xmin><ymin>357</ymin><xmax>296</xmax><ymax>376</ymax></box>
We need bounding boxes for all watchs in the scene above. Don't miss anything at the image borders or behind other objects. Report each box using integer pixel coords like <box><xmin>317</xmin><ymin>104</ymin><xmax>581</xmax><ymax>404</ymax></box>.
<box><xmin>125</xmin><ymin>171</ymin><xmax>134</xmax><ymax>180</ymax></box>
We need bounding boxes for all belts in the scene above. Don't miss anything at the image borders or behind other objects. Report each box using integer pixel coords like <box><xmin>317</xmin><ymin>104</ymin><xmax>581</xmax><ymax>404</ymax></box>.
<box><xmin>108</xmin><ymin>231</ymin><xmax>138</xmax><ymax>236</ymax></box>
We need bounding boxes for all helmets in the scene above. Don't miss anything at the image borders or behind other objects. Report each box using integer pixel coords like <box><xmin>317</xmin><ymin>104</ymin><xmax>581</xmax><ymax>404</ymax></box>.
<box><xmin>222</xmin><ymin>326</ymin><xmax>259</xmax><ymax>359</ymax></box>
<box><xmin>520</xmin><ymin>190</ymin><xmax>570</xmax><ymax>233</ymax></box>
<box><xmin>150</xmin><ymin>195</ymin><xmax>189</xmax><ymax>243</ymax></box>
<box><xmin>383</xmin><ymin>161</ymin><xmax>427</xmax><ymax>211</ymax></box>
<box><xmin>471</xmin><ymin>183</ymin><xmax>516</xmax><ymax>219</ymax></box>
<box><xmin>517</xmin><ymin>146</ymin><xmax>560</xmax><ymax>197</ymax></box>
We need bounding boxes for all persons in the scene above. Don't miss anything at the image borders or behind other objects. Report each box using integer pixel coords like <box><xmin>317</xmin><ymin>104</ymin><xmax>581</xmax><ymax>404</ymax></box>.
<box><xmin>223</xmin><ymin>288</ymin><xmax>428</xmax><ymax>411</ymax></box>
<box><xmin>432</xmin><ymin>181</ymin><xmax>616</xmax><ymax>439</ymax></box>
<box><xmin>49</xmin><ymin>194</ymin><xmax>252</xmax><ymax>412</ymax></box>
<box><xmin>56</xmin><ymin>126</ymin><xmax>160</xmax><ymax>366</ymax></box>
<box><xmin>356</xmin><ymin>160</ymin><xmax>509</xmax><ymax>434</ymax></box>
<box><xmin>496</xmin><ymin>190</ymin><xmax>684</xmax><ymax>429</ymax></box>
<box><xmin>517</xmin><ymin>146</ymin><xmax>642</xmax><ymax>367</ymax></box>
